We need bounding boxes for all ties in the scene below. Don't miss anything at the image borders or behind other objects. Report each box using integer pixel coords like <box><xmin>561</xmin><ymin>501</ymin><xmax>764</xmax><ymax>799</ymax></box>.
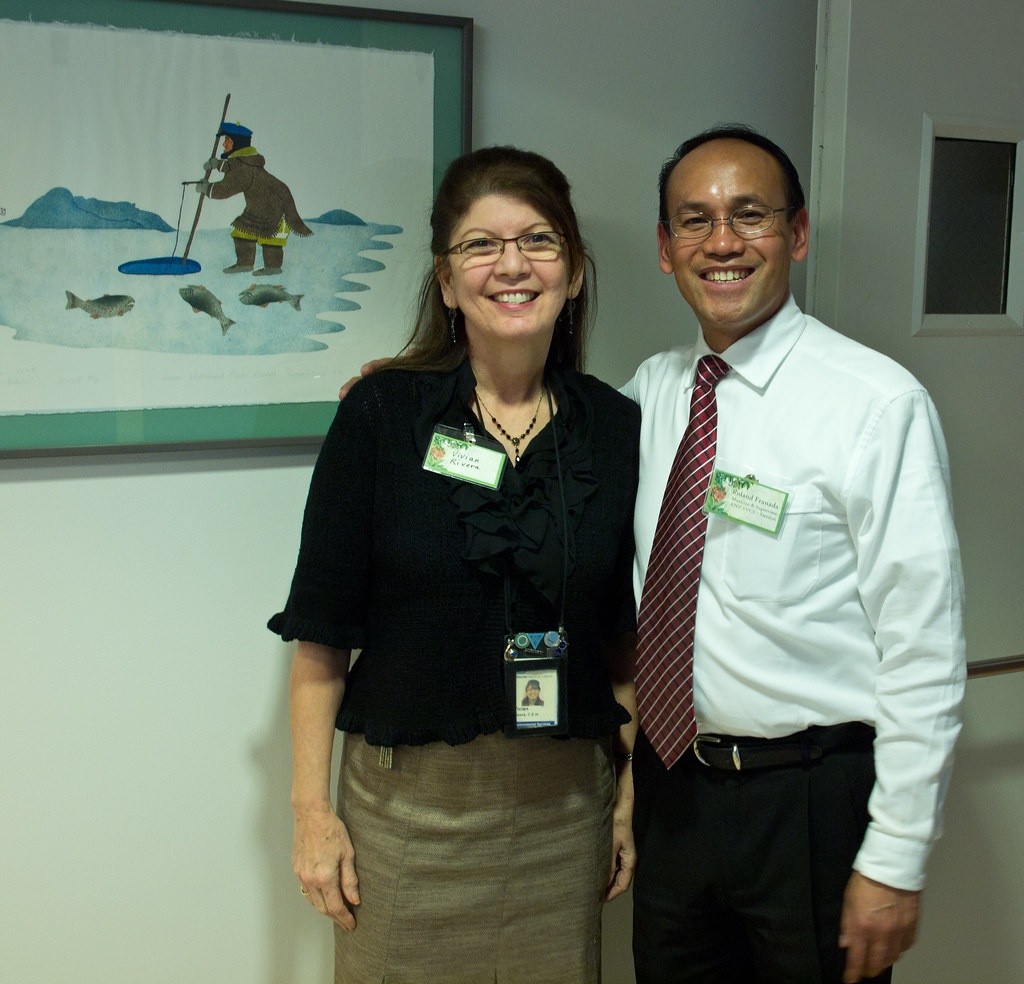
<box><xmin>635</xmin><ymin>354</ymin><xmax>729</xmax><ymax>769</ymax></box>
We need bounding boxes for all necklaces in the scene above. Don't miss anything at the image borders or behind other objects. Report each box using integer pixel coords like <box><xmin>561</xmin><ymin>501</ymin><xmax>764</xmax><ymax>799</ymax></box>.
<box><xmin>476</xmin><ymin>386</ymin><xmax>544</xmax><ymax>464</ymax></box>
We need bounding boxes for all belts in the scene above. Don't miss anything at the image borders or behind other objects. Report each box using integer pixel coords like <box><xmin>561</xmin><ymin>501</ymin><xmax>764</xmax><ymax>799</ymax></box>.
<box><xmin>685</xmin><ymin>722</ymin><xmax>873</xmax><ymax>771</ymax></box>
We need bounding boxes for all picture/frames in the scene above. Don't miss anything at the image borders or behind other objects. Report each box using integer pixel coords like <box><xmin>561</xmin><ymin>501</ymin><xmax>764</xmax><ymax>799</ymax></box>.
<box><xmin>0</xmin><ymin>0</ymin><xmax>476</xmax><ymax>458</ymax></box>
<box><xmin>912</xmin><ymin>112</ymin><xmax>1024</xmax><ymax>335</ymax></box>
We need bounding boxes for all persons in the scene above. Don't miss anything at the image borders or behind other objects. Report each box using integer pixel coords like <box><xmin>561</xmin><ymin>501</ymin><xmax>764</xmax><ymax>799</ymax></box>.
<box><xmin>264</xmin><ymin>147</ymin><xmax>641</xmax><ymax>984</ymax></box>
<box><xmin>337</xmin><ymin>127</ymin><xmax>967</xmax><ymax>984</ymax></box>
<box><xmin>522</xmin><ymin>680</ymin><xmax>544</xmax><ymax>706</ymax></box>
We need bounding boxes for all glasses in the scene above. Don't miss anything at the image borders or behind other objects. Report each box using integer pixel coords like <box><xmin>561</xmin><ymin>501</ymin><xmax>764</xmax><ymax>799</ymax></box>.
<box><xmin>660</xmin><ymin>203</ymin><xmax>799</xmax><ymax>239</ymax></box>
<box><xmin>442</xmin><ymin>230</ymin><xmax>568</xmax><ymax>264</ymax></box>
